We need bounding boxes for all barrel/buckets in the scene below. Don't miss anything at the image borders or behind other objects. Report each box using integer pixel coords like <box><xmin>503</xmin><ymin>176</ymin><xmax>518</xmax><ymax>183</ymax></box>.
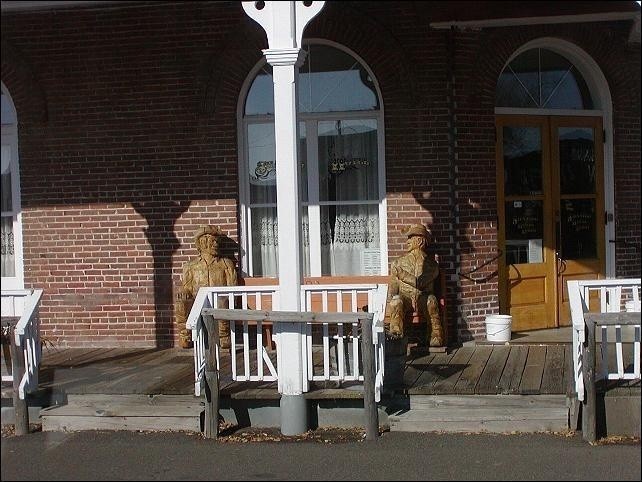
<box><xmin>481</xmin><ymin>311</ymin><xmax>514</xmax><ymax>341</ymax></box>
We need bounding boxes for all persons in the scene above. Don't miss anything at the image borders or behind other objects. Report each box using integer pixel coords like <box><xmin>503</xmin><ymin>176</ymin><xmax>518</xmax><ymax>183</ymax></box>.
<box><xmin>388</xmin><ymin>225</ymin><xmax>445</xmax><ymax>347</ymax></box>
<box><xmin>176</xmin><ymin>225</ymin><xmax>238</xmax><ymax>349</ymax></box>
